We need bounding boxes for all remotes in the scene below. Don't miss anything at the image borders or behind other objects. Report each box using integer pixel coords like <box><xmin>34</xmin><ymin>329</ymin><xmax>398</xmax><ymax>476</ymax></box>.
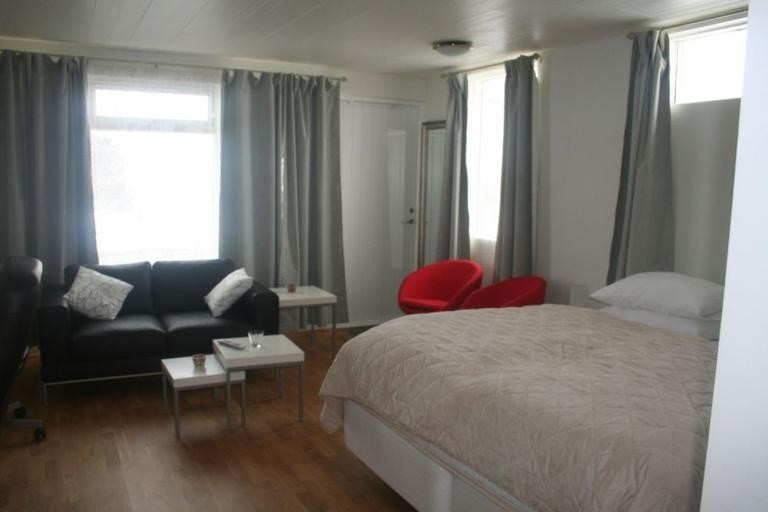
<box><xmin>218</xmin><ymin>339</ymin><xmax>245</xmax><ymax>350</ymax></box>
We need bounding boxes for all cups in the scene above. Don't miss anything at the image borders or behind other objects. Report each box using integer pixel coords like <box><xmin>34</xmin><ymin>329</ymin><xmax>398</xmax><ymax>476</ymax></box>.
<box><xmin>248</xmin><ymin>329</ymin><xmax>264</xmax><ymax>348</ymax></box>
<box><xmin>193</xmin><ymin>354</ymin><xmax>205</xmax><ymax>366</ymax></box>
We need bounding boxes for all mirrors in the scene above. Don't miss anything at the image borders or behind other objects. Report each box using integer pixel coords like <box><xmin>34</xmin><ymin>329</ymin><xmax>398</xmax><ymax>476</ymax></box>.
<box><xmin>420</xmin><ymin>121</ymin><xmax>448</xmax><ymax>271</ymax></box>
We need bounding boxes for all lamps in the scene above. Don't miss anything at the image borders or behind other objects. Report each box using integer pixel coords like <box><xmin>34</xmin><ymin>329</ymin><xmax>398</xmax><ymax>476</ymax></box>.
<box><xmin>434</xmin><ymin>43</ymin><xmax>474</xmax><ymax>57</ymax></box>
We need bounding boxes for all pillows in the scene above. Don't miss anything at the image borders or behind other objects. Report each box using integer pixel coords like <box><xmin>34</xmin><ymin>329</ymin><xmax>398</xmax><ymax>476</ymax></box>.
<box><xmin>591</xmin><ymin>272</ymin><xmax>724</xmax><ymax>321</ymax></box>
<box><xmin>602</xmin><ymin>307</ymin><xmax>721</xmax><ymax>340</ymax></box>
<box><xmin>204</xmin><ymin>267</ymin><xmax>254</xmax><ymax>315</ymax></box>
<box><xmin>62</xmin><ymin>266</ymin><xmax>133</xmax><ymax>320</ymax></box>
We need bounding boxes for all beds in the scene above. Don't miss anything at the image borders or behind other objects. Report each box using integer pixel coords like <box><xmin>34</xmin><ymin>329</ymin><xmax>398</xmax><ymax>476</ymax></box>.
<box><xmin>318</xmin><ymin>305</ymin><xmax>723</xmax><ymax>512</ymax></box>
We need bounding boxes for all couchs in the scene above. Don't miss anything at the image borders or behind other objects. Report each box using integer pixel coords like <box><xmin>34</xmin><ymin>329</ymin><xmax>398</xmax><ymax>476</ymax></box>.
<box><xmin>34</xmin><ymin>258</ymin><xmax>279</xmax><ymax>400</ymax></box>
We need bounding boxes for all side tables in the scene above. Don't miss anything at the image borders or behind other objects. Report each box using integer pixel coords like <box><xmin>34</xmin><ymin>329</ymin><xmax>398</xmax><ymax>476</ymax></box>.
<box><xmin>267</xmin><ymin>285</ymin><xmax>338</xmax><ymax>362</ymax></box>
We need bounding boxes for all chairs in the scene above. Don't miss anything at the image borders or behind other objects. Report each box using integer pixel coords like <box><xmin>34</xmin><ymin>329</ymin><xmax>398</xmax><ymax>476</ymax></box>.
<box><xmin>398</xmin><ymin>258</ymin><xmax>483</xmax><ymax>314</ymax></box>
<box><xmin>0</xmin><ymin>254</ymin><xmax>50</xmax><ymax>442</ymax></box>
<box><xmin>458</xmin><ymin>275</ymin><xmax>547</xmax><ymax>311</ymax></box>
